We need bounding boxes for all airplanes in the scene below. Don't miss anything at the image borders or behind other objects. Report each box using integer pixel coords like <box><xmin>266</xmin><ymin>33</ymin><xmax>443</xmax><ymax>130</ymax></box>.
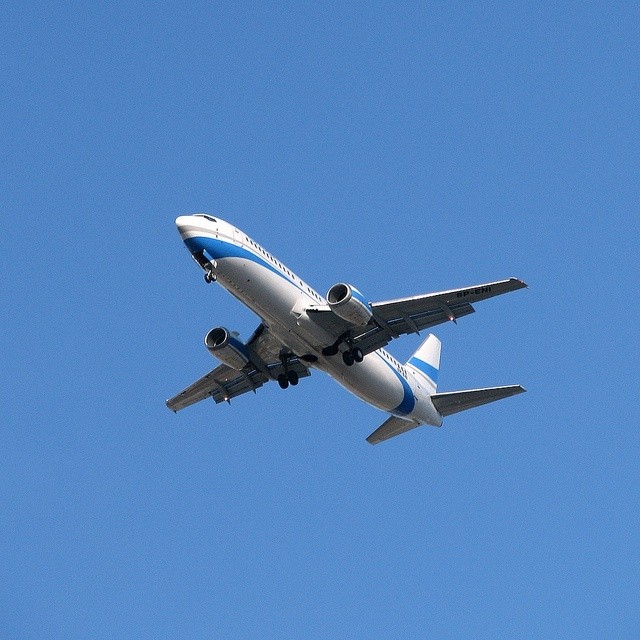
<box><xmin>165</xmin><ymin>214</ymin><xmax>528</xmax><ymax>445</ymax></box>
